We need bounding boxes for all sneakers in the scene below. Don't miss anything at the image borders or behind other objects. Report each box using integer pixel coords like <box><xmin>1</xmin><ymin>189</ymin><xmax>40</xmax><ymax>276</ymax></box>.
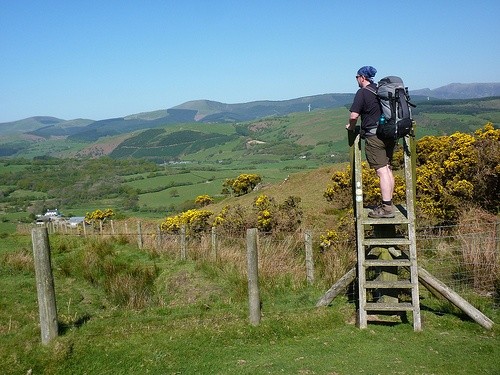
<box><xmin>368</xmin><ymin>201</ymin><xmax>395</xmax><ymax>218</ymax></box>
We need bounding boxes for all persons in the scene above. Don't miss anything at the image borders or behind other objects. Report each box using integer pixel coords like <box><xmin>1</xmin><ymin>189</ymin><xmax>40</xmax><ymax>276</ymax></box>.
<box><xmin>345</xmin><ymin>66</ymin><xmax>395</xmax><ymax>218</ymax></box>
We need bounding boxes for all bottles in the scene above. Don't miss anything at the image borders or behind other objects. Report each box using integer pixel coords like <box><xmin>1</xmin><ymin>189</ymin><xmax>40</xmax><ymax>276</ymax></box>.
<box><xmin>379</xmin><ymin>115</ymin><xmax>385</xmax><ymax>128</ymax></box>
<box><xmin>411</xmin><ymin>118</ymin><xmax>416</xmax><ymax>133</ymax></box>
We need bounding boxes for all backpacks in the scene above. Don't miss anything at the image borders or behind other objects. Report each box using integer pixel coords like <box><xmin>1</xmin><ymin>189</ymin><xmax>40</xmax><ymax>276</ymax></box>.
<box><xmin>366</xmin><ymin>76</ymin><xmax>412</xmax><ymax>139</ymax></box>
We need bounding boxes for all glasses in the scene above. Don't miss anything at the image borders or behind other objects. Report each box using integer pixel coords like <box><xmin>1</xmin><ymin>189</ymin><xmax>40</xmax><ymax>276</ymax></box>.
<box><xmin>355</xmin><ymin>75</ymin><xmax>362</xmax><ymax>78</ymax></box>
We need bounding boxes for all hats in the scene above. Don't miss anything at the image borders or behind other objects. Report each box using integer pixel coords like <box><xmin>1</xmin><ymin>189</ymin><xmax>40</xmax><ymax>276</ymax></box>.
<box><xmin>357</xmin><ymin>65</ymin><xmax>377</xmax><ymax>80</ymax></box>
